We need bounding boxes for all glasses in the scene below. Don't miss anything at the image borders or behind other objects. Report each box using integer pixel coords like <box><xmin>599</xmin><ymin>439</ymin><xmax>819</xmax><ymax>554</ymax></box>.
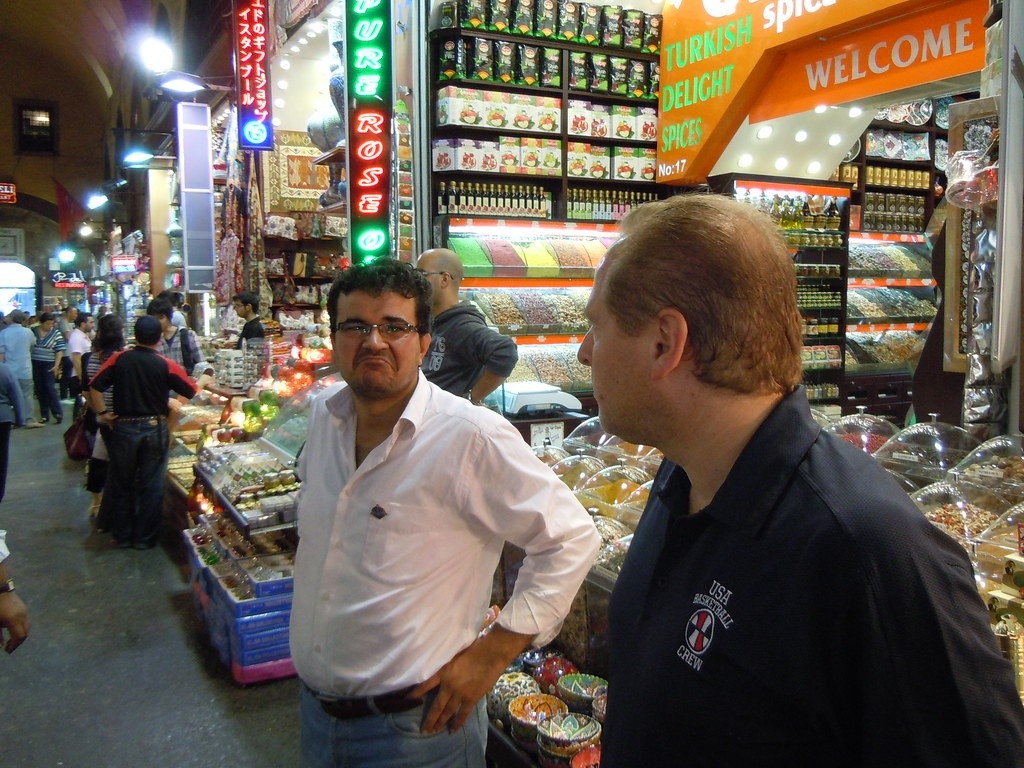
<box><xmin>233</xmin><ymin>304</ymin><xmax>246</xmax><ymax>308</ymax></box>
<box><xmin>334</xmin><ymin>320</ymin><xmax>423</xmax><ymax>340</ymax></box>
<box><xmin>419</xmin><ymin>269</ymin><xmax>455</xmax><ymax>281</ymax></box>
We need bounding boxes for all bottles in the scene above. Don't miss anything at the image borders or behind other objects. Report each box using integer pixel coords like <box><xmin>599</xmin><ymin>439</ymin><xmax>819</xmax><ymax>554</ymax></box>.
<box><xmin>437</xmin><ymin>181</ymin><xmax>548</xmax><ymax>219</ymax></box>
<box><xmin>802</xmin><ymin>378</ymin><xmax>839</xmax><ymax>399</ymax></box>
<box><xmin>567</xmin><ymin>188</ymin><xmax>660</xmax><ymax>220</ymax></box>
<box><xmin>740</xmin><ymin>189</ymin><xmax>843</xmax><ymax>337</ymax></box>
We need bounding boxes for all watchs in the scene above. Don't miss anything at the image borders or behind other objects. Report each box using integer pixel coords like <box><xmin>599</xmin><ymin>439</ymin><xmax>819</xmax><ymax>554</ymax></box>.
<box><xmin>0</xmin><ymin>579</ymin><xmax>15</xmax><ymax>594</ymax></box>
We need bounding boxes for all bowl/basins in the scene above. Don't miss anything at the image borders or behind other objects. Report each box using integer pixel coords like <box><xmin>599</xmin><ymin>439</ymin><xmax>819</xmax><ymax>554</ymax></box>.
<box><xmin>486</xmin><ymin>647</ymin><xmax>608</xmax><ymax>768</ymax></box>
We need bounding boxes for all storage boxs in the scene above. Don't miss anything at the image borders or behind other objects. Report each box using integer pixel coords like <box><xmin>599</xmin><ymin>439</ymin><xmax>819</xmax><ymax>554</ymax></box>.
<box><xmin>183</xmin><ymin>513</ymin><xmax>295</xmax><ymax>665</ymax></box>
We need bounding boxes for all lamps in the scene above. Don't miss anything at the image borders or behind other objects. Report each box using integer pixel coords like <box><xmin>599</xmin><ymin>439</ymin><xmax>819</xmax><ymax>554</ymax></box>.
<box><xmin>59</xmin><ymin>34</ymin><xmax>234</xmax><ymax>266</ymax></box>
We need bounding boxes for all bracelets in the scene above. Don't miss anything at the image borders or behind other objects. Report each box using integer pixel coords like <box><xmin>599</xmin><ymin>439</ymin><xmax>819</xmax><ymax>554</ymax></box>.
<box><xmin>98</xmin><ymin>409</ymin><xmax>107</xmax><ymax>415</ymax></box>
<box><xmin>467</xmin><ymin>389</ymin><xmax>482</xmax><ymax>406</ymax></box>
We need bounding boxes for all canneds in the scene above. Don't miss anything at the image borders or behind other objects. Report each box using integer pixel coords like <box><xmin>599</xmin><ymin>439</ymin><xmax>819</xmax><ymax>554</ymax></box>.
<box><xmin>236</xmin><ymin>470</ymin><xmax>303</xmax><ymax>512</ymax></box>
<box><xmin>792</xmin><ymin>262</ymin><xmax>841</xmax><ymax>338</ymax></box>
<box><xmin>831</xmin><ymin>164</ymin><xmax>930</xmax><ymax>234</ymax></box>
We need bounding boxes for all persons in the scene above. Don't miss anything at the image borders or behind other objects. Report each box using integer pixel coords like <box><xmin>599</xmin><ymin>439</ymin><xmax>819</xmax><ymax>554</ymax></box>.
<box><xmin>577</xmin><ymin>197</ymin><xmax>1024</xmax><ymax>768</ymax></box>
<box><xmin>414</xmin><ymin>249</ymin><xmax>518</xmax><ymax>406</ymax></box>
<box><xmin>288</xmin><ymin>257</ymin><xmax>602</xmax><ymax>768</ymax></box>
<box><xmin>0</xmin><ymin>288</ymin><xmax>213</xmax><ymax>653</ymax></box>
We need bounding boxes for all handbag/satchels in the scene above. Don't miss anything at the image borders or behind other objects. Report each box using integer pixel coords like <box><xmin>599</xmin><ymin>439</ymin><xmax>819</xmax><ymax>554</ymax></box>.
<box><xmin>63</xmin><ymin>406</ymin><xmax>92</xmax><ymax>460</ymax></box>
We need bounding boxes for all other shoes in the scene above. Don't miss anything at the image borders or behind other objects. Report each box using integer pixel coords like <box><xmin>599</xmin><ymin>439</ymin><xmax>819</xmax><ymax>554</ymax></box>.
<box><xmin>59</xmin><ymin>398</ymin><xmax>75</xmax><ymax>406</ymax></box>
<box><xmin>56</xmin><ymin>414</ymin><xmax>62</xmax><ymax>424</ymax></box>
<box><xmin>39</xmin><ymin>417</ymin><xmax>48</xmax><ymax>424</ymax></box>
<box><xmin>97</xmin><ymin>524</ymin><xmax>110</xmax><ymax>533</ymax></box>
<box><xmin>26</xmin><ymin>422</ymin><xmax>45</xmax><ymax>428</ymax></box>
<box><xmin>110</xmin><ymin>537</ymin><xmax>134</xmax><ymax>548</ymax></box>
<box><xmin>134</xmin><ymin>541</ymin><xmax>150</xmax><ymax>549</ymax></box>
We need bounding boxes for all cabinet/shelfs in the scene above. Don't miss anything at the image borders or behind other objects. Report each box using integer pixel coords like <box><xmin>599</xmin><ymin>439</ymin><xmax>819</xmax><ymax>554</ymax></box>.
<box><xmin>426</xmin><ymin>0</ymin><xmax>955</xmax><ymax>416</ymax></box>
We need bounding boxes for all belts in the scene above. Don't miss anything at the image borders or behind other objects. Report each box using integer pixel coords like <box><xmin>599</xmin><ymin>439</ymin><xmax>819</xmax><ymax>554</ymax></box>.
<box><xmin>115</xmin><ymin>414</ymin><xmax>168</xmax><ymax>423</ymax></box>
<box><xmin>306</xmin><ymin>685</ymin><xmax>425</xmax><ymax>717</ymax></box>
